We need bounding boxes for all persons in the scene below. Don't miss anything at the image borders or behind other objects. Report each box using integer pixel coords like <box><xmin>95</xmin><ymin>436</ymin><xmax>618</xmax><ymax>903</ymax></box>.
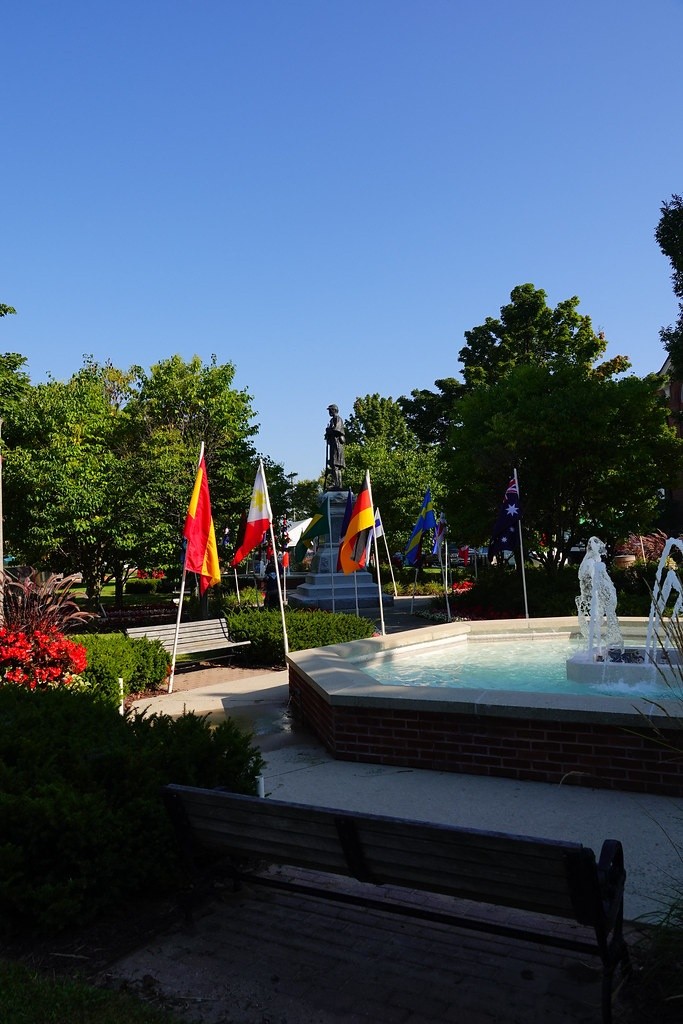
<box><xmin>324</xmin><ymin>404</ymin><xmax>347</xmax><ymax>489</ymax></box>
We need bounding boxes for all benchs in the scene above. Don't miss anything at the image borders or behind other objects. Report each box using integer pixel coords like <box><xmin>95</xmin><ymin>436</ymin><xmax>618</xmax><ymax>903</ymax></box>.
<box><xmin>124</xmin><ymin>619</ymin><xmax>253</xmax><ymax>668</ymax></box>
<box><xmin>160</xmin><ymin>784</ymin><xmax>637</xmax><ymax>1024</ymax></box>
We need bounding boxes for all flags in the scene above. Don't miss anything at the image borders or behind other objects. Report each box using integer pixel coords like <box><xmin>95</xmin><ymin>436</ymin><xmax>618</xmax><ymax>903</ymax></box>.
<box><xmin>268</xmin><ymin>489</ymin><xmax>448</xmax><ymax>575</ymax></box>
<box><xmin>458</xmin><ymin>546</ymin><xmax>469</xmax><ymax>566</ymax></box>
<box><xmin>492</xmin><ymin>477</ymin><xmax>523</xmax><ymax>550</ymax></box>
<box><xmin>183</xmin><ymin>455</ymin><xmax>221</xmax><ymax>596</ymax></box>
<box><xmin>229</xmin><ymin>463</ymin><xmax>272</xmax><ymax>566</ymax></box>
